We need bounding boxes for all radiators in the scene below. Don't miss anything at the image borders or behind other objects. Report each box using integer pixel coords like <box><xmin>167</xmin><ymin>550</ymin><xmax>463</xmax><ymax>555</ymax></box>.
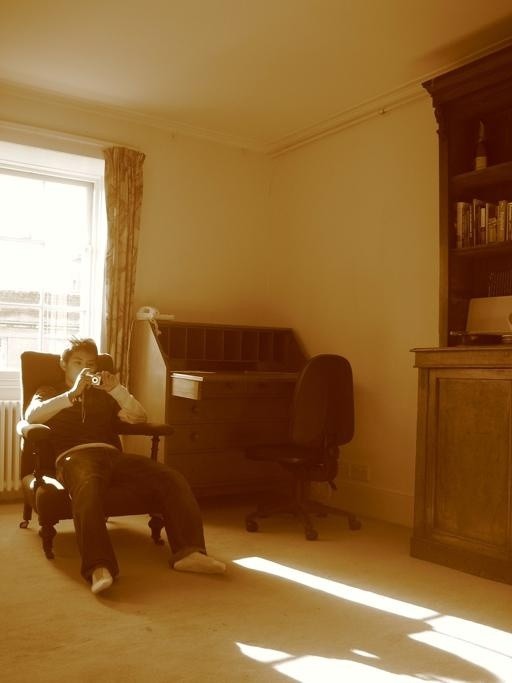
<box><xmin>1</xmin><ymin>399</ymin><xmax>28</xmax><ymax>494</ymax></box>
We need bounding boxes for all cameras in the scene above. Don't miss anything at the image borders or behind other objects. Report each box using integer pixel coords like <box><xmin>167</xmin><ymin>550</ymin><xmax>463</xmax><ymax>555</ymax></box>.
<box><xmin>85</xmin><ymin>372</ymin><xmax>102</xmax><ymax>386</ymax></box>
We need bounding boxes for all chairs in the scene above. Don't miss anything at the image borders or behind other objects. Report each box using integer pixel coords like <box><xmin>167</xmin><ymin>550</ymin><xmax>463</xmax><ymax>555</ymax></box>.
<box><xmin>245</xmin><ymin>353</ymin><xmax>362</xmax><ymax>540</ymax></box>
<box><xmin>19</xmin><ymin>350</ymin><xmax>173</xmax><ymax>561</ymax></box>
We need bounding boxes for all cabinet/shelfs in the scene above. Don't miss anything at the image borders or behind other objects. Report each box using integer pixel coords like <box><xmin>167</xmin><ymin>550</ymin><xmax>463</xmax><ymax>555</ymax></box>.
<box><xmin>129</xmin><ymin>318</ymin><xmax>327</xmax><ymax>520</ymax></box>
<box><xmin>407</xmin><ymin>46</ymin><xmax>511</xmax><ymax>590</ymax></box>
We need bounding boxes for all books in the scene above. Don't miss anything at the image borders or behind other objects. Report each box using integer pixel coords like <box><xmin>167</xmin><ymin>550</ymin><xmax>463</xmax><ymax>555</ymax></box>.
<box><xmin>453</xmin><ymin>195</ymin><xmax>512</xmax><ymax>249</ymax></box>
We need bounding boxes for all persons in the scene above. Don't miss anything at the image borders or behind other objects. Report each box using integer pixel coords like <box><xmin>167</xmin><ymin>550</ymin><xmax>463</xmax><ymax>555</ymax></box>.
<box><xmin>23</xmin><ymin>336</ymin><xmax>226</xmax><ymax>595</ymax></box>
